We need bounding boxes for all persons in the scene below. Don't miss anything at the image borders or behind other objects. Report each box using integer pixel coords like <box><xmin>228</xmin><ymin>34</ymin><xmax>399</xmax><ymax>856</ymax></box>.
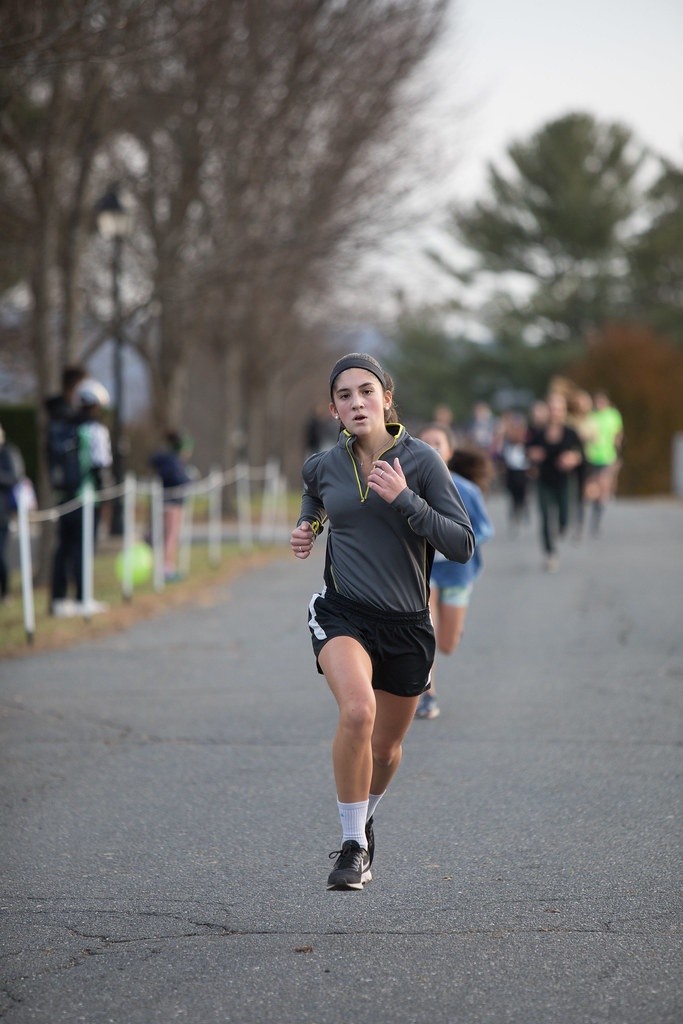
<box><xmin>1</xmin><ymin>366</ymin><xmax>201</xmax><ymax>622</ymax></box>
<box><xmin>416</xmin><ymin>375</ymin><xmax>626</xmax><ymax>719</ymax></box>
<box><xmin>289</xmin><ymin>353</ymin><xmax>477</xmax><ymax>890</ymax></box>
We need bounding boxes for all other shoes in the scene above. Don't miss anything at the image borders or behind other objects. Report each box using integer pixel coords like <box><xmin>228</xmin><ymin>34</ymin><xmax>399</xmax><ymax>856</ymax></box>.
<box><xmin>163</xmin><ymin>573</ymin><xmax>185</xmax><ymax>583</ymax></box>
<box><xmin>51</xmin><ymin>598</ymin><xmax>110</xmax><ymax>621</ymax></box>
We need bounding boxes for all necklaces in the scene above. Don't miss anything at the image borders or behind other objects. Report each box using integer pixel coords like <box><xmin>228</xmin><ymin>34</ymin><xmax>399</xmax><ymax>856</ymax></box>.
<box><xmin>350</xmin><ymin>436</ymin><xmax>395</xmax><ymax>467</ymax></box>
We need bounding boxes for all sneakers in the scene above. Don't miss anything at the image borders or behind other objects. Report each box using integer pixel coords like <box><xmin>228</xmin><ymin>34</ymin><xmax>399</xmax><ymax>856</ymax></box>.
<box><xmin>327</xmin><ymin>840</ymin><xmax>372</xmax><ymax>891</ymax></box>
<box><xmin>365</xmin><ymin>815</ymin><xmax>375</xmax><ymax>863</ymax></box>
<box><xmin>414</xmin><ymin>693</ymin><xmax>440</xmax><ymax>719</ymax></box>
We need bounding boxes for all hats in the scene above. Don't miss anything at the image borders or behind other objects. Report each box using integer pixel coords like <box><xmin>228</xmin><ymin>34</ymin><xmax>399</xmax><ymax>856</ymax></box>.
<box><xmin>72</xmin><ymin>381</ymin><xmax>110</xmax><ymax>406</ymax></box>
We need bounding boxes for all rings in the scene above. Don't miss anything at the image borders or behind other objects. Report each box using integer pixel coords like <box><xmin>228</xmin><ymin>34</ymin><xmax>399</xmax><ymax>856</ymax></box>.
<box><xmin>299</xmin><ymin>546</ymin><xmax>303</xmax><ymax>552</ymax></box>
<box><xmin>379</xmin><ymin>471</ymin><xmax>385</xmax><ymax>477</ymax></box>
